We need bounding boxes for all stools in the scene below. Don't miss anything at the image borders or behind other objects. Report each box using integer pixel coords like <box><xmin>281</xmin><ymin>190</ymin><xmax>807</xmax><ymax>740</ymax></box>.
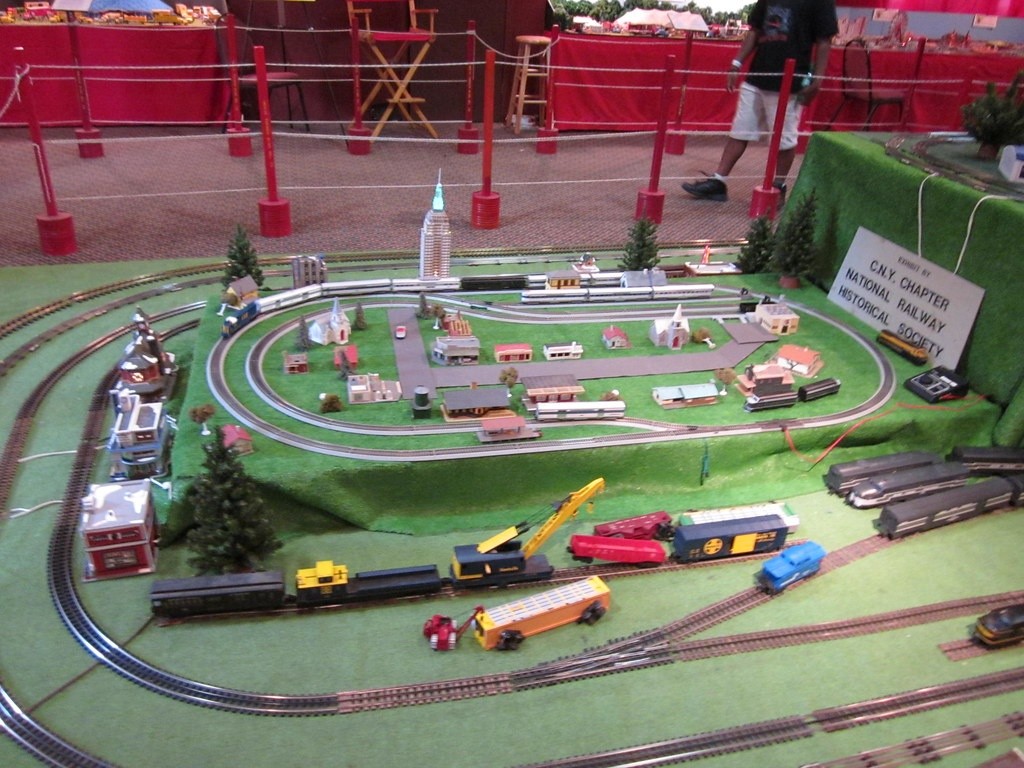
<box><xmin>504</xmin><ymin>36</ymin><xmax>550</xmax><ymax>135</ymax></box>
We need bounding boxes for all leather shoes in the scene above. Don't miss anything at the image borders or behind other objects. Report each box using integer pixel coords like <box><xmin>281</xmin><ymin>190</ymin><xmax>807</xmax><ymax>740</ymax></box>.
<box><xmin>774</xmin><ymin>182</ymin><xmax>786</xmax><ymax>211</ymax></box>
<box><xmin>681</xmin><ymin>179</ymin><xmax>727</xmax><ymax>202</ymax></box>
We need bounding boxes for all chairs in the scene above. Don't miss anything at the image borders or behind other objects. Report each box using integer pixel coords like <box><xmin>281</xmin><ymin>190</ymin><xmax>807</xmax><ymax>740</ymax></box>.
<box><xmin>823</xmin><ymin>38</ymin><xmax>905</xmax><ymax>130</ymax></box>
<box><xmin>346</xmin><ymin>0</ymin><xmax>438</xmax><ymax>143</ymax></box>
<box><xmin>215</xmin><ymin>12</ymin><xmax>311</xmax><ymax>134</ymax></box>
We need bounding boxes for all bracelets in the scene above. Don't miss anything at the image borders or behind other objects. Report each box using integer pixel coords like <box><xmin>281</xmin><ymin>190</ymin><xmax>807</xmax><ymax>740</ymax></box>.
<box><xmin>731</xmin><ymin>59</ymin><xmax>742</xmax><ymax>68</ymax></box>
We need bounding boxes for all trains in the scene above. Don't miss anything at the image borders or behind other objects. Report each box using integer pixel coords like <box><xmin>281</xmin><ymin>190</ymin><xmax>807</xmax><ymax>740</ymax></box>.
<box><xmin>150</xmin><ymin>476</ymin><xmax>608</xmax><ymax>619</ymax></box>
<box><xmin>826</xmin><ymin>444</ymin><xmax>1024</xmax><ymax>540</ymax></box>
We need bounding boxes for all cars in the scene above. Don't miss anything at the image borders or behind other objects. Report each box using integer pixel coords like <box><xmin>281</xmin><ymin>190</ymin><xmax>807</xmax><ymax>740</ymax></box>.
<box><xmin>972</xmin><ymin>601</ymin><xmax>1024</xmax><ymax>647</ymax></box>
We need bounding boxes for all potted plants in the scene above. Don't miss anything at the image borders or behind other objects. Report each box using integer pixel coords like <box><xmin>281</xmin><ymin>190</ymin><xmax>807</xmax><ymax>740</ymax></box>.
<box><xmin>156</xmin><ymin>423</ymin><xmax>284</xmax><ymax>579</ymax></box>
<box><xmin>763</xmin><ymin>184</ymin><xmax>822</xmax><ymax>288</ymax></box>
<box><xmin>961</xmin><ymin>80</ymin><xmax>1024</xmax><ymax>160</ymax></box>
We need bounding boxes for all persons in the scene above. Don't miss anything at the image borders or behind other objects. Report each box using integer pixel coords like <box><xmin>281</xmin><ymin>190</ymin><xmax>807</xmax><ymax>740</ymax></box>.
<box><xmin>681</xmin><ymin>0</ymin><xmax>839</xmax><ymax>209</ymax></box>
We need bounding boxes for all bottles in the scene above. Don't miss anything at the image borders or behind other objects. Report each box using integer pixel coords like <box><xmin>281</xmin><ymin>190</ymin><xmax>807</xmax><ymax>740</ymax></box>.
<box><xmin>797</xmin><ymin>64</ymin><xmax>817</xmax><ymax>106</ymax></box>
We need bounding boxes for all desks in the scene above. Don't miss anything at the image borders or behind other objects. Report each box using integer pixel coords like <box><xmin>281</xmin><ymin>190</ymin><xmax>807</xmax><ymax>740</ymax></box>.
<box><xmin>1</xmin><ymin>24</ymin><xmax>231</xmax><ymax>125</ymax></box>
<box><xmin>542</xmin><ymin>30</ymin><xmax>1024</xmax><ymax>131</ymax></box>
